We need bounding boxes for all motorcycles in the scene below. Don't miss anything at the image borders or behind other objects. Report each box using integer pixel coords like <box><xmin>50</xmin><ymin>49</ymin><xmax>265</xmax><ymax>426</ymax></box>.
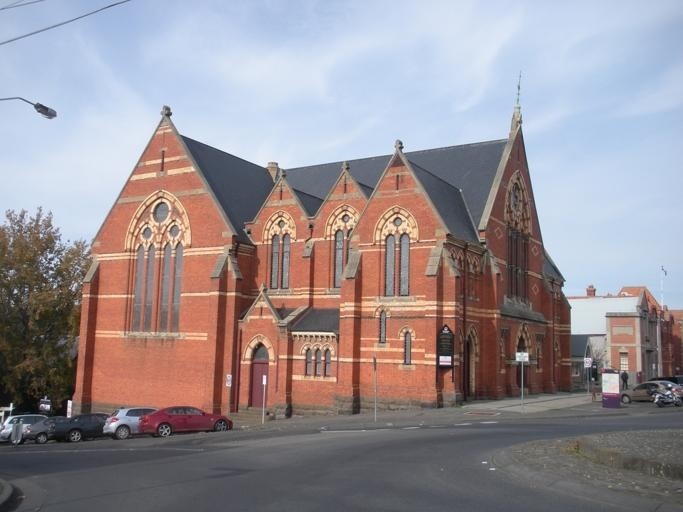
<box><xmin>648</xmin><ymin>385</ymin><xmax>683</xmax><ymax>408</ymax></box>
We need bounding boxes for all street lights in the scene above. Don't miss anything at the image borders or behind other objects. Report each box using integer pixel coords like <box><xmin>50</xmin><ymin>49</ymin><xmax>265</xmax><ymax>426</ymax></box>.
<box><xmin>0</xmin><ymin>95</ymin><xmax>56</xmax><ymax>120</ymax></box>
<box><xmin>657</xmin><ymin>264</ymin><xmax>669</xmax><ymax>311</ymax></box>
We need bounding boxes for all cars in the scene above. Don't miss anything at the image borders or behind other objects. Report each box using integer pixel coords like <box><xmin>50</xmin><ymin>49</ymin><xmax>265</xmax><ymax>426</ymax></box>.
<box><xmin>620</xmin><ymin>380</ymin><xmax>682</xmax><ymax>404</ymax></box>
<box><xmin>649</xmin><ymin>376</ymin><xmax>683</xmax><ymax>387</ymax></box>
<box><xmin>0</xmin><ymin>406</ymin><xmax>234</xmax><ymax>443</ymax></box>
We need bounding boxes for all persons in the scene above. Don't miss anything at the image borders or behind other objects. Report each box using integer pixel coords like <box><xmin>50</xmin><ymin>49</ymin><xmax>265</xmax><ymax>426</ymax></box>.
<box><xmin>621</xmin><ymin>369</ymin><xmax>628</xmax><ymax>390</ymax></box>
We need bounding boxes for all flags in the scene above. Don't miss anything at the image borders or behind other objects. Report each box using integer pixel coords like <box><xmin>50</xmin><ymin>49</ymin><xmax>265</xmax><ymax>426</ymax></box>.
<box><xmin>661</xmin><ymin>265</ymin><xmax>667</xmax><ymax>276</ymax></box>
